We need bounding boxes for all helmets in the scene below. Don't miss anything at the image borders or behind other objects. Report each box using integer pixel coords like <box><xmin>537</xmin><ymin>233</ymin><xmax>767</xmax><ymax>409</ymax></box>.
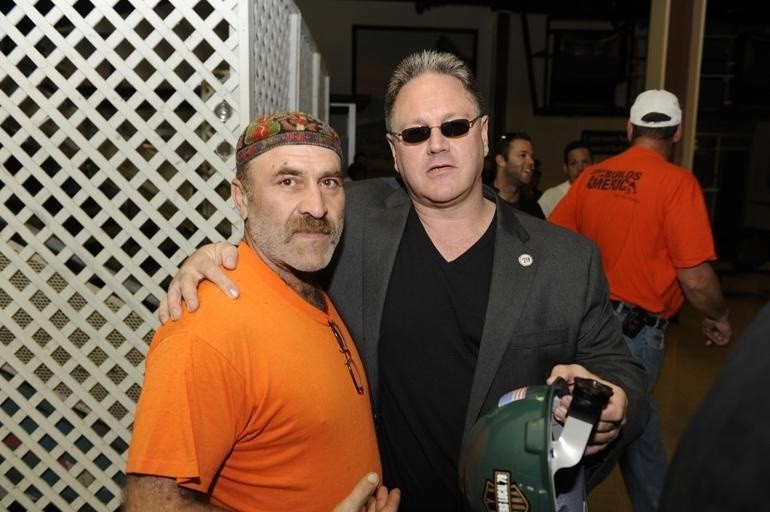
<box><xmin>458</xmin><ymin>378</ymin><xmax>587</xmax><ymax>512</ymax></box>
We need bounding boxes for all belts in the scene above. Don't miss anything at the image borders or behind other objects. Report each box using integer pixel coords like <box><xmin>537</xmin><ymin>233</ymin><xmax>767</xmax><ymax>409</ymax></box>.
<box><xmin>611</xmin><ymin>298</ymin><xmax>667</xmax><ymax>331</ymax></box>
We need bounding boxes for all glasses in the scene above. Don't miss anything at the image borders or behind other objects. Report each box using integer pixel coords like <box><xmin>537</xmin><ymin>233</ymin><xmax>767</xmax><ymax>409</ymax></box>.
<box><xmin>390</xmin><ymin>115</ymin><xmax>481</xmax><ymax>144</ymax></box>
<box><xmin>328</xmin><ymin>318</ymin><xmax>365</xmax><ymax>395</ymax></box>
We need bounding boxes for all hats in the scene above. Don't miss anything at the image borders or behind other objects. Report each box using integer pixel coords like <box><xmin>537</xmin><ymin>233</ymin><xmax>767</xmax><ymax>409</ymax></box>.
<box><xmin>236</xmin><ymin>112</ymin><xmax>342</xmax><ymax>168</ymax></box>
<box><xmin>630</xmin><ymin>89</ymin><xmax>681</xmax><ymax>129</ymax></box>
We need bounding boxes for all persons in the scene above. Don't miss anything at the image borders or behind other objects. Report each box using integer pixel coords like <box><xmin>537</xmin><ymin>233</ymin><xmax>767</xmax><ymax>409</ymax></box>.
<box><xmin>488</xmin><ymin>131</ymin><xmax>547</xmax><ymax>221</ymax></box>
<box><xmin>121</xmin><ymin>108</ymin><xmax>405</xmax><ymax>510</ymax></box>
<box><xmin>657</xmin><ymin>305</ymin><xmax>770</xmax><ymax>511</ymax></box>
<box><xmin>545</xmin><ymin>85</ymin><xmax>735</xmax><ymax>511</ymax></box>
<box><xmin>538</xmin><ymin>138</ymin><xmax>594</xmax><ymax>223</ymax></box>
<box><xmin>156</xmin><ymin>43</ymin><xmax>653</xmax><ymax>511</ymax></box>
